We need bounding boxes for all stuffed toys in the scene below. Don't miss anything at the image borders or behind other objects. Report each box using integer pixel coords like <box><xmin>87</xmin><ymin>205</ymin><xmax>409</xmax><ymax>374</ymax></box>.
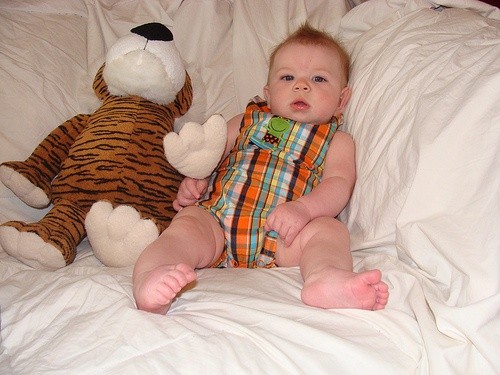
<box><xmin>1</xmin><ymin>21</ymin><xmax>228</xmax><ymax>272</ymax></box>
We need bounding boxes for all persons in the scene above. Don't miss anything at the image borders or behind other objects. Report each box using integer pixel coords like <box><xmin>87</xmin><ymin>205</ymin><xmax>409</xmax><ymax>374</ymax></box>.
<box><xmin>130</xmin><ymin>27</ymin><xmax>390</xmax><ymax>315</ymax></box>
